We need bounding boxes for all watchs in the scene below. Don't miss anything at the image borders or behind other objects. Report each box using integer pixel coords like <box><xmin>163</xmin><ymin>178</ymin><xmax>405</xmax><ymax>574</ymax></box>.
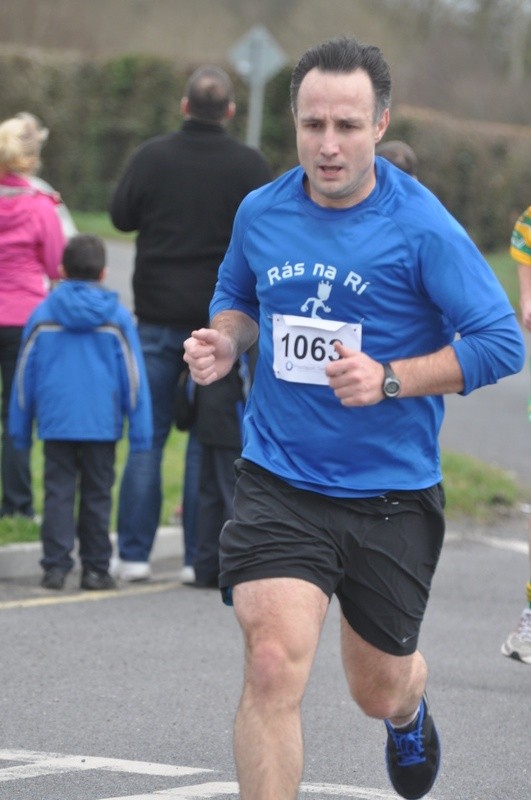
<box><xmin>380</xmin><ymin>363</ymin><xmax>402</xmax><ymax>400</ymax></box>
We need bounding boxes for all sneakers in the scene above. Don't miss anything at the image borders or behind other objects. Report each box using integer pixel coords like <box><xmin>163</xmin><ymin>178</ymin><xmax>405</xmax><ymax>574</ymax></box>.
<box><xmin>500</xmin><ymin>608</ymin><xmax>531</xmax><ymax>663</ymax></box>
<box><xmin>382</xmin><ymin>687</ymin><xmax>442</xmax><ymax>799</ymax></box>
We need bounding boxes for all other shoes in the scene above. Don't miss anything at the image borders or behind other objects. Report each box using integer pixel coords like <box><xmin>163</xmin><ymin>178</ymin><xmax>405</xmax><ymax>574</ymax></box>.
<box><xmin>79</xmin><ymin>568</ymin><xmax>116</xmax><ymax>590</ymax></box>
<box><xmin>39</xmin><ymin>572</ymin><xmax>65</xmax><ymax>589</ymax></box>
<box><xmin>179</xmin><ymin>565</ymin><xmax>198</xmax><ymax>584</ymax></box>
<box><xmin>106</xmin><ymin>555</ymin><xmax>152</xmax><ymax>582</ymax></box>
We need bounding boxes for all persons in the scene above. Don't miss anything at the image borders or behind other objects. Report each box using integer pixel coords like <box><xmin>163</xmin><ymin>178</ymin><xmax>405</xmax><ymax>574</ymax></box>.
<box><xmin>10</xmin><ymin>233</ymin><xmax>154</xmax><ymax>592</ymax></box>
<box><xmin>501</xmin><ymin>204</ymin><xmax>531</xmax><ymax>665</ymax></box>
<box><xmin>376</xmin><ymin>139</ymin><xmax>420</xmax><ymax>181</ymax></box>
<box><xmin>0</xmin><ymin>109</ymin><xmax>77</xmax><ymax>524</ymax></box>
<box><xmin>108</xmin><ymin>64</ymin><xmax>272</xmax><ymax>587</ymax></box>
<box><xmin>183</xmin><ymin>37</ymin><xmax>524</xmax><ymax>800</ymax></box>
<box><xmin>175</xmin><ymin>332</ymin><xmax>255</xmax><ymax>591</ymax></box>
